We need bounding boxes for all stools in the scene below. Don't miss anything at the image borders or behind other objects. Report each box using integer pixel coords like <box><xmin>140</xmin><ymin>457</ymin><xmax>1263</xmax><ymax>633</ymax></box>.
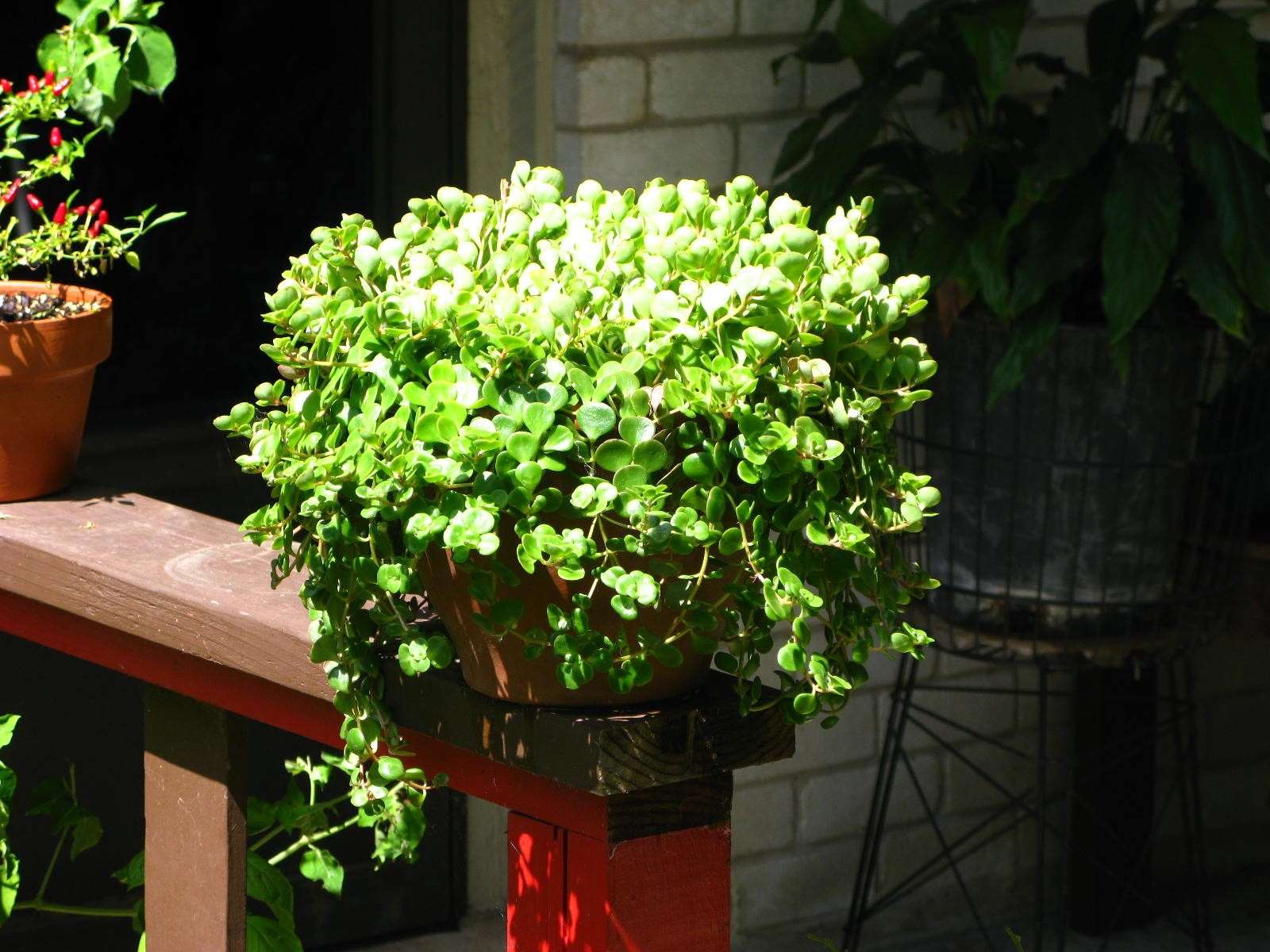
<box><xmin>841</xmin><ymin>603</ymin><xmax>1199</xmax><ymax>952</ymax></box>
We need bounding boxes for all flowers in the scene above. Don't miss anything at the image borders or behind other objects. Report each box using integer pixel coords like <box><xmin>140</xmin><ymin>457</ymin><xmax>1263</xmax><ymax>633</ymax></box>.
<box><xmin>0</xmin><ymin>0</ymin><xmax>188</xmax><ymax>322</ymax></box>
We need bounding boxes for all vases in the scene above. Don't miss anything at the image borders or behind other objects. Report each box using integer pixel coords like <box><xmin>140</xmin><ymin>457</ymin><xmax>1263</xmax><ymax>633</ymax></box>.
<box><xmin>0</xmin><ymin>280</ymin><xmax>114</xmax><ymax>504</ymax></box>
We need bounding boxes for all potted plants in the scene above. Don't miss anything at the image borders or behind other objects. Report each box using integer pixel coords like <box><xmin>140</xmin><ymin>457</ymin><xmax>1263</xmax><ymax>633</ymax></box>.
<box><xmin>209</xmin><ymin>161</ymin><xmax>942</xmax><ymax>896</ymax></box>
<box><xmin>756</xmin><ymin>0</ymin><xmax>1270</xmax><ymax>562</ymax></box>
